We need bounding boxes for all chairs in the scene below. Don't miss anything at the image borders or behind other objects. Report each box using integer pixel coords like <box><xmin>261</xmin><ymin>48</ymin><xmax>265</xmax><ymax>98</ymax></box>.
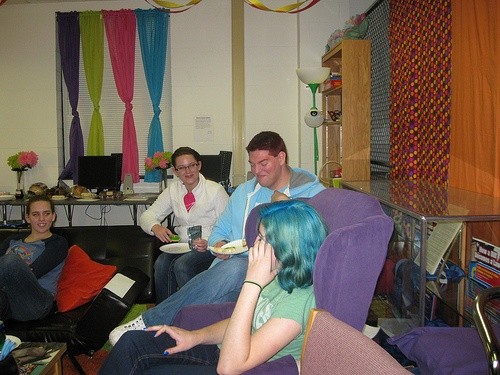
<box><xmin>171</xmin><ymin>187</ymin><xmax>394</xmax><ymax>375</ymax></box>
<box><xmin>300</xmin><ymin>308</ymin><xmax>414</xmax><ymax>375</ymax></box>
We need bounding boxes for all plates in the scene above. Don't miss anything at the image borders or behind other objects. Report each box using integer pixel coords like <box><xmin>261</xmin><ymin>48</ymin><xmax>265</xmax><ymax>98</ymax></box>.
<box><xmin>208</xmin><ymin>239</ymin><xmax>249</xmax><ymax>254</ymax></box>
<box><xmin>159</xmin><ymin>243</ymin><xmax>191</xmax><ymax>254</ymax></box>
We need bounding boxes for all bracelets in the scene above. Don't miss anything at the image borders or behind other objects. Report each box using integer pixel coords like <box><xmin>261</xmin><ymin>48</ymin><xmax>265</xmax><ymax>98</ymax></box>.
<box><xmin>242</xmin><ymin>281</ymin><xmax>263</xmax><ymax>291</ymax></box>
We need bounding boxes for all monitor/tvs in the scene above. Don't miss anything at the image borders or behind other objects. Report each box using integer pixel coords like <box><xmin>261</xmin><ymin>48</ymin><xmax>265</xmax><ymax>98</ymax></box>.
<box><xmin>78</xmin><ymin>156</ymin><xmax>120</xmax><ymax>195</ymax></box>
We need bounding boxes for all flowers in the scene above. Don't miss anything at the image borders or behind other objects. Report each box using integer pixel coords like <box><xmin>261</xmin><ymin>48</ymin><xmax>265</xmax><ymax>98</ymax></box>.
<box><xmin>144</xmin><ymin>152</ymin><xmax>172</xmax><ymax>172</ymax></box>
<box><xmin>7</xmin><ymin>150</ymin><xmax>39</xmax><ymax>169</ymax></box>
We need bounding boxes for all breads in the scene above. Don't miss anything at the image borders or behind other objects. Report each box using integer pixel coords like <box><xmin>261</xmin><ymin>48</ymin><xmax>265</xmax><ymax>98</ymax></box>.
<box><xmin>72</xmin><ymin>186</ymin><xmax>89</xmax><ymax>197</ymax></box>
<box><xmin>29</xmin><ymin>182</ymin><xmax>49</xmax><ymax>195</ymax></box>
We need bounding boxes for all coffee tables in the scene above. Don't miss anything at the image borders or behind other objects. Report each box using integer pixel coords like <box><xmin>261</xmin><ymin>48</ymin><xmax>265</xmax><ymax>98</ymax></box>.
<box><xmin>11</xmin><ymin>342</ymin><xmax>67</xmax><ymax>375</ymax></box>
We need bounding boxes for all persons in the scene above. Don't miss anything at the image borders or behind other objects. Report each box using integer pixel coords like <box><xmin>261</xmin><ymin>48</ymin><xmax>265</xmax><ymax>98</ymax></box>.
<box><xmin>108</xmin><ymin>130</ymin><xmax>326</xmax><ymax>348</ymax></box>
<box><xmin>138</xmin><ymin>146</ymin><xmax>233</xmax><ymax>305</ymax></box>
<box><xmin>1</xmin><ymin>194</ymin><xmax>69</xmax><ymax>362</ymax></box>
<box><xmin>97</xmin><ymin>200</ymin><xmax>329</xmax><ymax>375</ymax></box>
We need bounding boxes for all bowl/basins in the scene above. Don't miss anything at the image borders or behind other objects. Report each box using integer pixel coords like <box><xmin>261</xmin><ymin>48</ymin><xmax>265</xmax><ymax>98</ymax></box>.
<box><xmin>0</xmin><ymin>195</ymin><xmax>14</xmax><ymax>201</ymax></box>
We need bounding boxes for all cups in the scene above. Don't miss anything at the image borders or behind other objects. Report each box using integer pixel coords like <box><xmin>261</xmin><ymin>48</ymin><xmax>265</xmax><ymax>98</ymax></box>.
<box><xmin>188</xmin><ymin>225</ymin><xmax>202</xmax><ymax>252</ymax></box>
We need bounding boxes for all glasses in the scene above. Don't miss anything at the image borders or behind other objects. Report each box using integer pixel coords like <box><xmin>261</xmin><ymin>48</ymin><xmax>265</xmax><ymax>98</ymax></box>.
<box><xmin>175</xmin><ymin>163</ymin><xmax>199</xmax><ymax>172</ymax></box>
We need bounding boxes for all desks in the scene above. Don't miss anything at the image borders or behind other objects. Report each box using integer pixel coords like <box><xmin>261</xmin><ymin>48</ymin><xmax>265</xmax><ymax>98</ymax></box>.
<box><xmin>0</xmin><ymin>193</ymin><xmax>161</xmax><ymax>227</ymax></box>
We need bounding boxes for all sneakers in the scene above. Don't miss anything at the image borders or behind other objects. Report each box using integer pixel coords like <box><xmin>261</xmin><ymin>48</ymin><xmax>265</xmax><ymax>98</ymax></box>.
<box><xmin>109</xmin><ymin>315</ymin><xmax>147</xmax><ymax>346</ymax></box>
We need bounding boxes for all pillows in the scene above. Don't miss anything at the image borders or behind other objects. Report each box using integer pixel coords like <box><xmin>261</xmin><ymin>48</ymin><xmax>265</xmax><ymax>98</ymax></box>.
<box><xmin>387</xmin><ymin>326</ymin><xmax>500</xmax><ymax>375</ymax></box>
<box><xmin>56</xmin><ymin>245</ymin><xmax>116</xmax><ymax>313</ymax></box>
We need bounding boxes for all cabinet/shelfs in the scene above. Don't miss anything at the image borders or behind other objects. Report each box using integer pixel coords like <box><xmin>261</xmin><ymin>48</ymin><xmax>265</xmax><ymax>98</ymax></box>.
<box><xmin>321</xmin><ymin>39</ymin><xmax>370</xmax><ymax>186</ymax></box>
<box><xmin>340</xmin><ymin>179</ymin><xmax>500</xmax><ymax>375</ymax></box>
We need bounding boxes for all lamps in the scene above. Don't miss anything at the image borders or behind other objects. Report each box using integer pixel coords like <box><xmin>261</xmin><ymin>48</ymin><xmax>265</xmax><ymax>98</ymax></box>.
<box><xmin>296</xmin><ymin>68</ymin><xmax>331</xmax><ymax>175</ymax></box>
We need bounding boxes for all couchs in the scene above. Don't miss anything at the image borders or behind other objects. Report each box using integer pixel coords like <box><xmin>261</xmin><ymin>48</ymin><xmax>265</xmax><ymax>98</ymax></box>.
<box><xmin>0</xmin><ymin>226</ymin><xmax>166</xmax><ymax>355</ymax></box>
